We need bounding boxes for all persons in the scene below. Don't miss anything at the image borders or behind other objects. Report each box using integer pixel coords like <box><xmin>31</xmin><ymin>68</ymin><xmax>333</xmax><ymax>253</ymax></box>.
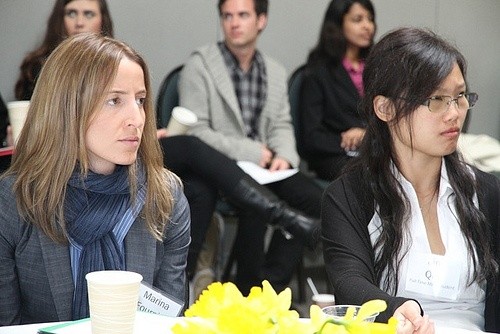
<box><xmin>321</xmin><ymin>29</ymin><xmax>500</xmax><ymax>334</ymax></box>
<box><xmin>15</xmin><ymin>0</ymin><xmax>322</xmax><ymax>280</ymax></box>
<box><xmin>178</xmin><ymin>0</ymin><xmax>323</xmax><ymax>297</ymax></box>
<box><xmin>298</xmin><ymin>0</ymin><xmax>377</xmax><ymax>180</ymax></box>
<box><xmin>0</xmin><ymin>33</ymin><xmax>191</xmax><ymax>328</ymax></box>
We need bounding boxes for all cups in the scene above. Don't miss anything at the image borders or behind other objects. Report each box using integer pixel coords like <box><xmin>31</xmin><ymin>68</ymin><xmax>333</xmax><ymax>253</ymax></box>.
<box><xmin>6</xmin><ymin>100</ymin><xmax>31</xmax><ymax>144</ymax></box>
<box><xmin>321</xmin><ymin>305</ymin><xmax>379</xmax><ymax>322</ymax></box>
<box><xmin>85</xmin><ymin>270</ymin><xmax>143</xmax><ymax>334</ymax></box>
<box><xmin>166</xmin><ymin>106</ymin><xmax>197</xmax><ymax>137</ymax></box>
<box><xmin>312</xmin><ymin>294</ymin><xmax>335</xmax><ymax>309</ymax></box>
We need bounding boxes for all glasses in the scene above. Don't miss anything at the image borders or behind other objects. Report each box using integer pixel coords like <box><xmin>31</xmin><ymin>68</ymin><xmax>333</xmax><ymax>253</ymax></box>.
<box><xmin>389</xmin><ymin>92</ymin><xmax>478</xmax><ymax>114</ymax></box>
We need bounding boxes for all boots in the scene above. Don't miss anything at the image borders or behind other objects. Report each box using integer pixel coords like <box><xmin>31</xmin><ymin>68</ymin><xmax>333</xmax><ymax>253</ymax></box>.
<box><xmin>227</xmin><ymin>173</ymin><xmax>323</xmax><ymax>251</ymax></box>
